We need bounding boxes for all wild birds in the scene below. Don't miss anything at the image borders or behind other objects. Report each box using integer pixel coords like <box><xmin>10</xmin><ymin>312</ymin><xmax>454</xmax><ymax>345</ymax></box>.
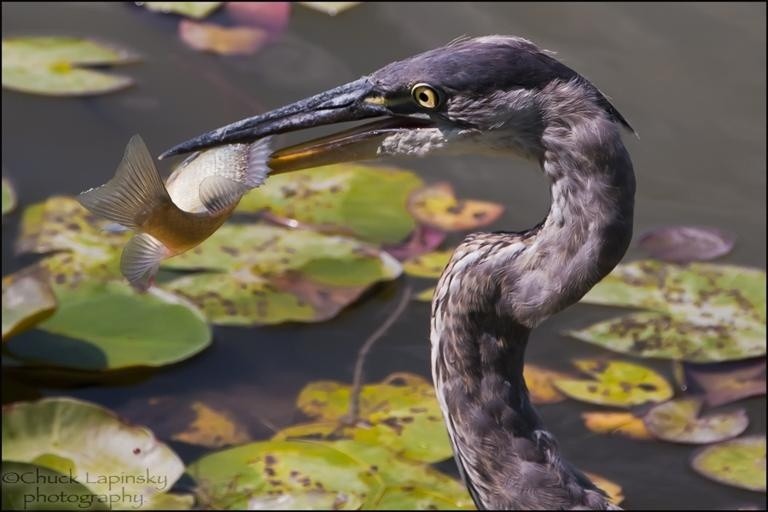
<box><xmin>157</xmin><ymin>32</ymin><xmax>640</xmax><ymax>510</ymax></box>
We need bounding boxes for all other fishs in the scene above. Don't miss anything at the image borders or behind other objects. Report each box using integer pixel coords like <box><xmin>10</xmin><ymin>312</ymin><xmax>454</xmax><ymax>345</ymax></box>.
<box><xmin>73</xmin><ymin>131</ymin><xmax>274</xmax><ymax>297</ymax></box>
